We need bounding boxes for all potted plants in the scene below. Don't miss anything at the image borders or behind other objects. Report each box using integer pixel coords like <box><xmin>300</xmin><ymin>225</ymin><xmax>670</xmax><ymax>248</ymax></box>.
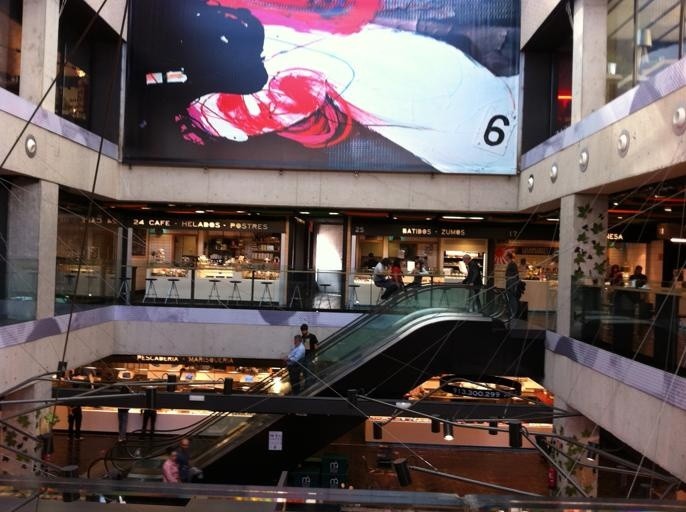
<box><xmin>608</xmin><ymin>46</ymin><xmax>620</xmax><ymax>74</ymax></box>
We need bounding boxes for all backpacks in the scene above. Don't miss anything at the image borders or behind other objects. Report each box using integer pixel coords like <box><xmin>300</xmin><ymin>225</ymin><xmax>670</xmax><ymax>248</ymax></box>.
<box><xmin>517</xmin><ymin>279</ymin><xmax>526</xmax><ymax>300</ymax></box>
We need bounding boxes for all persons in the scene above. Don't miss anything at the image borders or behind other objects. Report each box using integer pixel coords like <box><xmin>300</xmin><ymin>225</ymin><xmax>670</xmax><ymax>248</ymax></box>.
<box><xmin>300</xmin><ymin>324</ymin><xmax>321</xmax><ymax>389</ymax></box>
<box><xmin>176</xmin><ymin>437</ymin><xmax>197</xmax><ymax>482</ymax></box>
<box><xmin>161</xmin><ymin>446</ymin><xmax>181</xmax><ymax>483</ymax></box>
<box><xmin>140</xmin><ymin>378</ymin><xmax>159</xmax><ymax>440</ymax></box>
<box><xmin>99</xmin><ymin>474</ymin><xmax>127</xmax><ymax>505</ymax></box>
<box><xmin>366</xmin><ymin>250</ymin><xmax>648</xmax><ymax>322</ymax></box>
<box><xmin>118</xmin><ymin>386</ymin><xmax>130</xmax><ymax>442</ymax></box>
<box><xmin>175</xmin><ymin>367</ymin><xmax>189</xmax><ymax>386</ymax></box>
<box><xmin>66</xmin><ymin>383</ymin><xmax>87</xmax><ymax>442</ymax></box>
<box><xmin>284</xmin><ymin>334</ymin><xmax>305</xmax><ymax>393</ymax></box>
<box><xmin>225</xmin><ymin>250</ymin><xmax>245</xmax><ymax>264</ymax></box>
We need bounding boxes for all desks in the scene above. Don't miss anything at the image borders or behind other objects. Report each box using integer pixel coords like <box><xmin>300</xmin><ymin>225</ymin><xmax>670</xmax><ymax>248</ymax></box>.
<box><xmin>606</xmin><ymin>74</ymin><xmax>623</xmax><ymax>102</ymax></box>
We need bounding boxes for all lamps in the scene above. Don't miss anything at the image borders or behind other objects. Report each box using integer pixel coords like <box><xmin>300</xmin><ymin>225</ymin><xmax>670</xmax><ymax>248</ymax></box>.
<box><xmin>534</xmin><ymin>436</ymin><xmax>547</xmax><ymax>455</ymax></box>
<box><xmin>395</xmin><ymin>458</ymin><xmax>413</xmax><ymax>488</ymax></box>
<box><xmin>488</xmin><ymin>421</ymin><xmax>499</xmax><ymax>435</ymax></box>
<box><xmin>637</xmin><ymin>27</ymin><xmax>653</xmax><ymax>66</ymax></box>
<box><xmin>166</xmin><ymin>374</ymin><xmax>176</xmax><ymax>392</ymax></box>
<box><xmin>441</xmin><ymin>420</ymin><xmax>454</xmax><ymax>442</ymax></box>
<box><xmin>586</xmin><ymin>441</ymin><xmax>599</xmax><ymax>460</ymax></box>
<box><xmin>431</xmin><ymin>417</ymin><xmax>441</xmax><ymax>434</ymax></box>
<box><xmin>373</xmin><ymin>420</ymin><xmax>382</xmax><ymax>439</ymax></box>
<box><xmin>224</xmin><ymin>378</ymin><xmax>233</xmax><ymax>395</ymax></box>
<box><xmin>56</xmin><ymin>361</ymin><xmax>66</xmax><ymax>377</ymax></box>
<box><xmin>144</xmin><ymin>386</ymin><xmax>158</xmax><ymax>411</ymax></box>
<box><xmin>508</xmin><ymin>420</ymin><xmax>522</xmax><ymax>448</ymax></box>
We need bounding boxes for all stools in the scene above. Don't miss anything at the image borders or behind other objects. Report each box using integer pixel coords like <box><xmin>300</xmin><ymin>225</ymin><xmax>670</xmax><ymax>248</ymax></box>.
<box><xmin>375</xmin><ymin>286</ymin><xmax>388</xmax><ymax>304</ymax></box>
<box><xmin>289</xmin><ymin>282</ymin><xmax>306</xmax><ymax>309</ymax></box>
<box><xmin>259</xmin><ymin>281</ymin><xmax>273</xmax><ymax>308</ymax></box>
<box><xmin>116</xmin><ymin>278</ymin><xmax>132</xmax><ymax>304</ymax></box>
<box><xmin>229</xmin><ymin>281</ymin><xmax>243</xmax><ymax>304</ymax></box>
<box><xmin>348</xmin><ymin>285</ymin><xmax>360</xmax><ymax>309</ymax></box>
<box><xmin>209</xmin><ymin>280</ymin><xmax>221</xmax><ymax>302</ymax></box>
<box><xmin>143</xmin><ymin>278</ymin><xmax>158</xmax><ymax>303</ymax></box>
<box><xmin>317</xmin><ymin>283</ymin><xmax>332</xmax><ymax>309</ymax></box>
<box><xmin>165</xmin><ymin>279</ymin><xmax>179</xmax><ymax>304</ymax></box>
<box><xmin>438</xmin><ymin>286</ymin><xmax>451</xmax><ymax>307</ymax></box>
